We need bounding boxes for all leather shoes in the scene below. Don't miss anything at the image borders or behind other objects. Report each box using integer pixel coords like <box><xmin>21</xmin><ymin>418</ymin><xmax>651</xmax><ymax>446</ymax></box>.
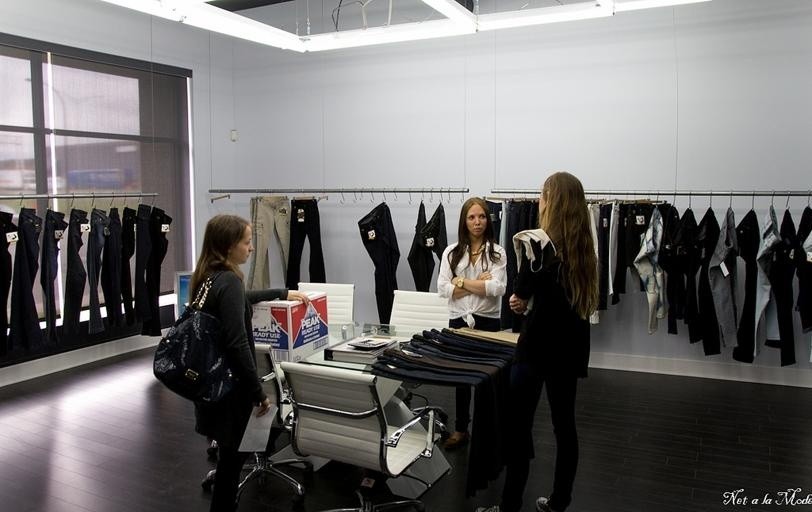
<box><xmin>442</xmin><ymin>430</ymin><xmax>469</xmax><ymax>451</ymax></box>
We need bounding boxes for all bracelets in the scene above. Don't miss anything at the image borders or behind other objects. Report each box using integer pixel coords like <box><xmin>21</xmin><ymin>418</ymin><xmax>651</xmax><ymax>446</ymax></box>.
<box><xmin>456</xmin><ymin>276</ymin><xmax>466</xmax><ymax>288</ymax></box>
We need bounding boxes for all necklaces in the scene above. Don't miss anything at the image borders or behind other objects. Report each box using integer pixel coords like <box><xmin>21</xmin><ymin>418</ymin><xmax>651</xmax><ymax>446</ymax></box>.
<box><xmin>471</xmin><ymin>249</ymin><xmax>481</xmax><ymax>256</ymax></box>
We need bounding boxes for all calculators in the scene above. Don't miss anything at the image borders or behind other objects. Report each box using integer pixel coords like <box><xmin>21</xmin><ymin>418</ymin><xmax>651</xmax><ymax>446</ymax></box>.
<box><xmin>346</xmin><ymin>340</ymin><xmax>389</xmax><ymax>350</ymax></box>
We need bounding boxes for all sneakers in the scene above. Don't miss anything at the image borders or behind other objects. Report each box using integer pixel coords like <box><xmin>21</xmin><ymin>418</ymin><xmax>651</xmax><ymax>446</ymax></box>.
<box><xmin>475</xmin><ymin>505</ymin><xmax>501</xmax><ymax>512</ymax></box>
<box><xmin>536</xmin><ymin>497</ymin><xmax>552</xmax><ymax>512</ymax></box>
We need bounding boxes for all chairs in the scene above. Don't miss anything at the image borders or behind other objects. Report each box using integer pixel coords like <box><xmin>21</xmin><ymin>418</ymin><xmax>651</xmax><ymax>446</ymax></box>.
<box><xmin>387</xmin><ymin>289</ymin><xmax>452</xmax><ymax>344</ymax></box>
<box><xmin>297</xmin><ymin>282</ymin><xmax>356</xmax><ymax>326</ymax></box>
<box><xmin>199</xmin><ymin>343</ymin><xmax>453</xmax><ymax>512</ymax></box>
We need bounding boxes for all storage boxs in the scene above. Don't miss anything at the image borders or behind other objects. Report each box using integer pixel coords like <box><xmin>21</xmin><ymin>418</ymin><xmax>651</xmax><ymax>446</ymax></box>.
<box><xmin>246</xmin><ymin>290</ymin><xmax>328</xmax><ymax>364</ymax></box>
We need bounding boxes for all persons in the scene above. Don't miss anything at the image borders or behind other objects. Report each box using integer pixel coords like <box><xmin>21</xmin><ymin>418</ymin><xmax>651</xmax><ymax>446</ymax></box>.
<box><xmin>437</xmin><ymin>197</ymin><xmax>508</xmax><ymax>448</ymax></box>
<box><xmin>190</xmin><ymin>213</ymin><xmax>310</xmax><ymax>511</ymax></box>
<box><xmin>471</xmin><ymin>171</ymin><xmax>598</xmax><ymax>512</ymax></box>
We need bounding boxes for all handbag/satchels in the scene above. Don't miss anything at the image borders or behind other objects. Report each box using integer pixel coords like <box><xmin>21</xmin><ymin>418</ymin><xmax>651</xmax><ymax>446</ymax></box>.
<box><xmin>152</xmin><ymin>266</ymin><xmax>243</xmax><ymax>409</ymax></box>
